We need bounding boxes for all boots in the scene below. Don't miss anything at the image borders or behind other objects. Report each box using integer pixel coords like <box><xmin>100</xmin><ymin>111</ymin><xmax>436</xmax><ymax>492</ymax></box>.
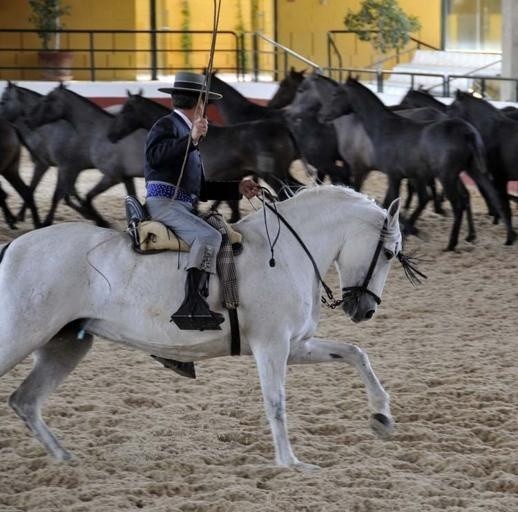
<box><xmin>181</xmin><ymin>268</ymin><xmax>222</xmax><ymax>317</ymax></box>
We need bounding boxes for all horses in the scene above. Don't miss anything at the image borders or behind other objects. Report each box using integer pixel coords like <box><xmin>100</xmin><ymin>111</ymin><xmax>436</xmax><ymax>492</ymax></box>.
<box><xmin>0</xmin><ymin>66</ymin><xmax>517</xmax><ymax>254</ymax></box>
<box><xmin>0</xmin><ymin>180</ymin><xmax>405</xmax><ymax>471</ymax></box>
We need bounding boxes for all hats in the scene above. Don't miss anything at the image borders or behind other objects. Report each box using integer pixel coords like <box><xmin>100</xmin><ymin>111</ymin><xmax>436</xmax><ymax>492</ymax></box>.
<box><xmin>159</xmin><ymin>72</ymin><xmax>223</xmax><ymax>100</ymax></box>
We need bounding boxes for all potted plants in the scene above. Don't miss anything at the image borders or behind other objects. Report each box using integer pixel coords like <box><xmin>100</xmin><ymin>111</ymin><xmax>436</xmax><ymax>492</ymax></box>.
<box><xmin>26</xmin><ymin>0</ymin><xmax>76</xmax><ymax>80</ymax></box>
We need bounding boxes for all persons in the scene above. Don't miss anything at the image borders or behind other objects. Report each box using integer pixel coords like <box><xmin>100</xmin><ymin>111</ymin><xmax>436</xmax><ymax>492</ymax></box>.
<box><xmin>143</xmin><ymin>71</ymin><xmax>262</xmax><ymax>325</ymax></box>
